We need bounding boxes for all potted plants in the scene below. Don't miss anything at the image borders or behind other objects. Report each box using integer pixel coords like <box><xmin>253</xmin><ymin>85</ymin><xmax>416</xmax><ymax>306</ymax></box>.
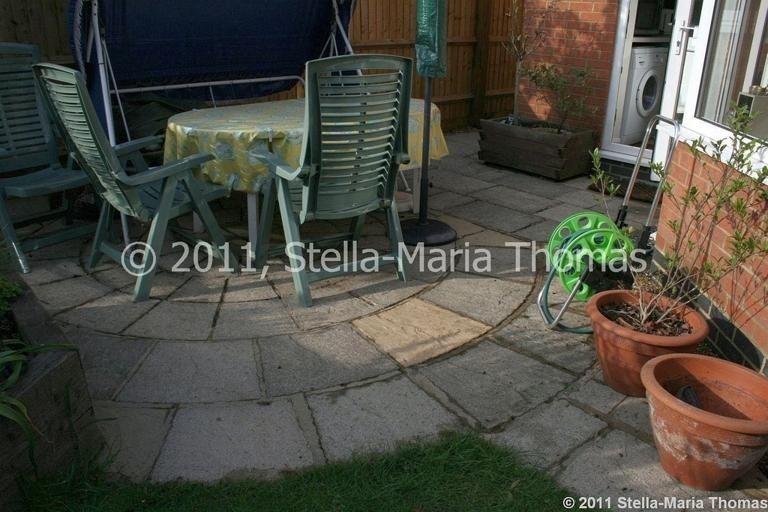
<box><xmin>585</xmin><ymin>99</ymin><xmax>768</xmax><ymax>399</ymax></box>
<box><xmin>477</xmin><ymin>1</ymin><xmax>603</xmax><ymax>183</ymax></box>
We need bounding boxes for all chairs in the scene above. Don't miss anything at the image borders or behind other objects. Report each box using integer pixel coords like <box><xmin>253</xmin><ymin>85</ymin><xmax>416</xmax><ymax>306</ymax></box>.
<box><xmin>258</xmin><ymin>53</ymin><xmax>416</xmax><ymax>307</ymax></box>
<box><xmin>30</xmin><ymin>59</ymin><xmax>243</xmax><ymax>306</ymax></box>
<box><xmin>0</xmin><ymin>41</ymin><xmax>99</xmax><ymax>275</ymax></box>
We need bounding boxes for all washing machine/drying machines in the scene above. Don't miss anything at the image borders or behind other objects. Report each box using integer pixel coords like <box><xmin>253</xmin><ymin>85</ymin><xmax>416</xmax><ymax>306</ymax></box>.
<box><xmin>621</xmin><ymin>46</ymin><xmax>668</xmax><ymax>145</ymax></box>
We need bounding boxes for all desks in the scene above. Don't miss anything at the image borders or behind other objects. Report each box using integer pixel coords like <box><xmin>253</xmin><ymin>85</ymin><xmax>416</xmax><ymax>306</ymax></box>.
<box><xmin>162</xmin><ymin>93</ymin><xmax>450</xmax><ymax>260</ymax></box>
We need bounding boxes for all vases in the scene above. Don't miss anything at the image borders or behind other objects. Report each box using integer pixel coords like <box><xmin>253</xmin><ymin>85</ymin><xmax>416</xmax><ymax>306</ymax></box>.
<box><xmin>639</xmin><ymin>351</ymin><xmax>768</xmax><ymax>494</ymax></box>
